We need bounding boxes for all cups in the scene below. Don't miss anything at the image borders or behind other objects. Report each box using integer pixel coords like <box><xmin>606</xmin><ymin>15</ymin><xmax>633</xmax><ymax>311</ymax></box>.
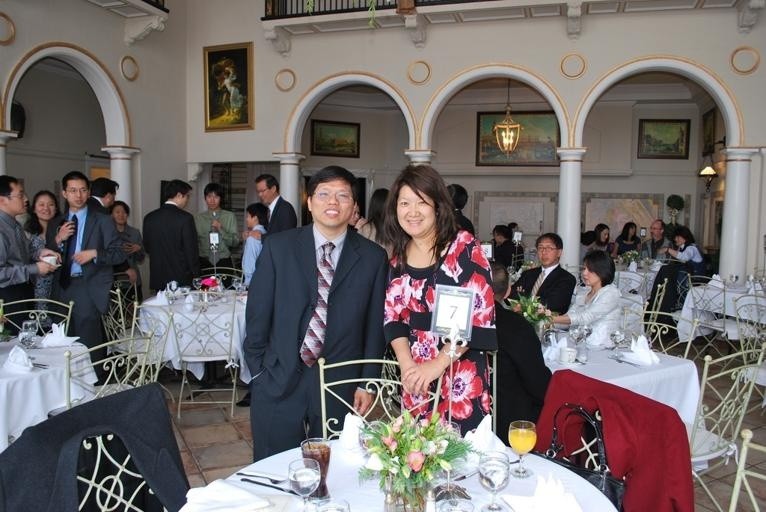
<box><xmin>209</xmin><ymin>206</ymin><xmax>221</xmax><ymax>230</ymax></box>
<box><xmin>23</xmin><ymin>320</ymin><xmax>38</xmax><ymax>349</ymax></box>
<box><xmin>435</xmin><ymin>420</ymin><xmax>460</xmax><ymax>479</ymax></box>
<box><xmin>169</xmin><ymin>282</ymin><xmax>178</xmax><ymax>301</ymax></box>
<box><xmin>509</xmin><ymin>420</ymin><xmax>536</xmax><ymax>479</ymax></box>
<box><xmin>236</xmin><ymin>284</ymin><xmax>247</xmax><ymax>305</ymax></box>
<box><xmin>193</xmin><ymin>279</ymin><xmax>202</xmax><ymax>291</ymax></box>
<box><xmin>560</xmin><ymin>345</ymin><xmax>575</xmax><ymax>363</ymax></box>
<box><xmin>441</xmin><ymin>498</ymin><xmax>473</xmax><ymax>512</ymax></box>
<box><xmin>315</xmin><ymin>499</ymin><xmax>351</xmax><ymax>511</ymax></box>
<box><xmin>232</xmin><ymin>278</ymin><xmax>242</xmax><ymax>292</ymax></box>
<box><xmin>301</xmin><ymin>438</ymin><xmax>332</xmax><ymax>503</ymax></box>
<box><xmin>288</xmin><ymin>458</ymin><xmax>320</xmax><ymax>510</ymax></box>
<box><xmin>43</xmin><ymin>256</ymin><xmax>57</xmax><ymax>265</ymax></box>
<box><xmin>19</xmin><ymin>330</ymin><xmax>36</xmax><ymax>361</ymax></box>
<box><xmin>478</xmin><ymin>451</ymin><xmax>511</xmax><ymax>512</ymax></box>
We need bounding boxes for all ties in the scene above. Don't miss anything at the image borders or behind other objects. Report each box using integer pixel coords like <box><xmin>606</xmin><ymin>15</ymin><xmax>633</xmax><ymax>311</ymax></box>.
<box><xmin>531</xmin><ymin>271</ymin><xmax>545</xmax><ymax>296</ymax></box>
<box><xmin>59</xmin><ymin>214</ymin><xmax>78</xmax><ymax>284</ymax></box>
<box><xmin>300</xmin><ymin>242</ymin><xmax>336</xmax><ymax>369</ymax></box>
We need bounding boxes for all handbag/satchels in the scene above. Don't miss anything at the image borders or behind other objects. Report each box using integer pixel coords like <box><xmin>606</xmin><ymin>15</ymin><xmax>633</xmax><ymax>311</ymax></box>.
<box><xmin>533</xmin><ymin>402</ymin><xmax>624</xmax><ymax>512</ymax></box>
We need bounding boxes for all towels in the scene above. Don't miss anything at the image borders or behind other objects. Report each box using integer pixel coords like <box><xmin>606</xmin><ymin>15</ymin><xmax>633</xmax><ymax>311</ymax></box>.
<box><xmin>144</xmin><ymin>288</ymin><xmax>169</xmax><ymax>305</ymax></box>
<box><xmin>622</xmin><ymin>334</ymin><xmax>660</xmax><ymax>366</ymax></box>
<box><xmin>184</xmin><ymin>295</ymin><xmax>193</xmax><ymax>311</ymax></box>
<box><xmin>42</xmin><ymin>322</ymin><xmax>78</xmax><ymax>347</ymax></box>
<box><xmin>542</xmin><ymin>338</ymin><xmax>568</xmax><ymax>361</ymax></box>
<box><xmin>709</xmin><ymin>274</ymin><xmax>723</xmax><ymax>288</ymax></box>
<box><xmin>465</xmin><ymin>414</ymin><xmax>503</xmax><ymax>467</ymax></box>
<box><xmin>4</xmin><ymin>344</ymin><xmax>33</xmax><ymax>372</ymax></box>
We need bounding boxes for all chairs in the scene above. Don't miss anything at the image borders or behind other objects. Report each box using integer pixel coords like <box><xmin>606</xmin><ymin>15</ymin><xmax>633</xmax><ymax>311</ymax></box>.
<box><xmin>165</xmin><ymin>292</ymin><xmax>240</xmax><ymax>420</ymax></box>
<box><xmin>508</xmin><ymin>250</ymin><xmax>538</xmax><ymax>291</ymax></box>
<box><xmin>48</xmin><ymin>329</ymin><xmax>154</xmax><ymax>421</ymax></box>
<box><xmin>566</xmin><ymin>264</ymin><xmax>586</xmax><ymax>290</ymax></box>
<box><xmin>0</xmin><ymin>383</ymin><xmax>190</xmax><ymax>512</ymax></box>
<box><xmin>534</xmin><ymin>370</ymin><xmax>681</xmax><ymax>512</ymax></box>
<box><xmin>107</xmin><ymin>279</ymin><xmax>140</xmax><ymax>340</ymax></box>
<box><xmin>93</xmin><ymin>303</ymin><xmax>174</xmax><ymax>398</ymax></box>
<box><xmin>100</xmin><ymin>288</ymin><xmax>174</xmax><ymax>404</ymax></box>
<box><xmin>686</xmin><ymin>273</ymin><xmax>758</xmax><ymax>365</ymax></box>
<box><xmin>644</xmin><ymin>278</ymin><xmax>668</xmax><ymax>338</ymax></box>
<box><xmin>689</xmin><ymin>344</ymin><xmax>766</xmax><ymax>512</ymax></box>
<box><xmin>730</xmin><ymin>427</ymin><xmax>765</xmax><ymax>512</ymax></box>
<box><xmin>623</xmin><ymin>308</ymin><xmax>698</xmax><ymax>358</ymax></box>
<box><xmin>193</xmin><ymin>267</ymin><xmax>246</xmax><ymax>291</ymax></box>
<box><xmin>318</xmin><ymin>358</ymin><xmax>444</xmax><ymax>442</ymax></box>
<box><xmin>0</xmin><ymin>298</ymin><xmax>74</xmax><ymax>337</ymax></box>
<box><xmin>733</xmin><ymin>294</ymin><xmax>766</xmax><ymax>417</ymax></box>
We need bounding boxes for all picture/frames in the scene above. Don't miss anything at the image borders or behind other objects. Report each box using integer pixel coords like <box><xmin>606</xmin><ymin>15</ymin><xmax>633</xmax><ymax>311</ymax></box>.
<box><xmin>202</xmin><ymin>42</ymin><xmax>254</xmax><ymax>132</ymax></box>
<box><xmin>311</xmin><ymin>119</ymin><xmax>361</xmax><ymax>159</ymax></box>
<box><xmin>476</xmin><ymin>109</ymin><xmax>561</xmax><ymax>166</ymax></box>
<box><xmin>702</xmin><ymin>106</ymin><xmax>717</xmax><ymax>158</ymax></box>
<box><xmin>636</xmin><ymin>117</ymin><xmax>691</xmax><ymax>159</ymax></box>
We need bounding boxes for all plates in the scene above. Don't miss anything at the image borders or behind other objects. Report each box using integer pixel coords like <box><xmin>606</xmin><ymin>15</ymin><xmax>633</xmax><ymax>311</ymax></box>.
<box><xmin>54</xmin><ymin>263</ymin><xmax>61</xmax><ymax>268</ymax></box>
<box><xmin>558</xmin><ymin>361</ymin><xmax>579</xmax><ymax>366</ymax></box>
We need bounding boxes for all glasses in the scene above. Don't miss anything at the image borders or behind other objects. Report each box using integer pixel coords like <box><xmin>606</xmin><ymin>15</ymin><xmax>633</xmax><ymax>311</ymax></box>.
<box><xmin>312</xmin><ymin>189</ymin><xmax>353</xmax><ymax>203</ymax></box>
<box><xmin>257</xmin><ymin>187</ymin><xmax>269</xmax><ymax>193</ymax></box>
<box><xmin>538</xmin><ymin>246</ymin><xmax>558</xmax><ymax>252</ymax></box>
<box><xmin>8</xmin><ymin>192</ymin><xmax>23</xmax><ymax>198</ymax></box>
<box><xmin>66</xmin><ymin>187</ymin><xmax>89</xmax><ymax>194</ymax></box>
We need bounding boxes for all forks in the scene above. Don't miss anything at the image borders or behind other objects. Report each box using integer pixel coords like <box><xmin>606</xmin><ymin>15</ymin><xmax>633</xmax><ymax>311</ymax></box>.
<box><xmin>236</xmin><ymin>472</ymin><xmax>290</xmax><ymax>485</ymax></box>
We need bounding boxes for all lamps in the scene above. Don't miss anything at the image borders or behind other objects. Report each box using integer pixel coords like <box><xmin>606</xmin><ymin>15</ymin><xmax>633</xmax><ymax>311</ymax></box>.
<box><xmin>492</xmin><ymin>105</ymin><xmax>524</xmax><ymax>158</ymax></box>
<box><xmin>696</xmin><ymin>137</ymin><xmax>727</xmax><ymax>194</ymax></box>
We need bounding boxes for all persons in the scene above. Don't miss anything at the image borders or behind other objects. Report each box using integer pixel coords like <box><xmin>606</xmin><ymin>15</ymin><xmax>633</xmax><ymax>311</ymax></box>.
<box><xmin>194</xmin><ymin>182</ymin><xmax>241</xmax><ymax>290</ymax></box>
<box><xmin>52</xmin><ymin>172</ymin><xmax>126</xmax><ymax>383</ymax></box>
<box><xmin>356</xmin><ymin>188</ymin><xmax>400</xmax><ymax>259</ymax></box>
<box><xmin>489</xmin><ymin>263</ymin><xmax>552</xmax><ymax>447</ymax></box>
<box><xmin>490</xmin><ymin>225</ymin><xmax>503</xmax><ymax>248</ymax></box>
<box><xmin>496</xmin><ymin>227</ymin><xmax>524</xmax><ymax>268</ymax></box>
<box><xmin>245</xmin><ymin>175</ymin><xmax>296</xmax><ymax>239</ymax></box>
<box><xmin>385</xmin><ymin>166</ymin><xmax>496</xmax><ymax>442</ymax></box>
<box><xmin>642</xmin><ymin>220</ymin><xmax>672</xmax><ymax>264</ymax></box>
<box><xmin>611</xmin><ymin>222</ymin><xmax>640</xmax><ymax>256</ymax></box>
<box><xmin>590</xmin><ymin>223</ymin><xmax>613</xmax><ymax>256</ymax></box>
<box><xmin>447</xmin><ymin>184</ymin><xmax>475</xmax><ymax>238</ymax></box>
<box><xmin>504</xmin><ymin>234</ymin><xmax>575</xmax><ymax>318</ymax></box>
<box><xmin>552</xmin><ymin>250</ymin><xmax>624</xmax><ymax>349</ymax></box>
<box><xmin>0</xmin><ymin>176</ymin><xmax>61</xmax><ymax>337</ymax></box>
<box><xmin>658</xmin><ymin>226</ymin><xmax>706</xmax><ymax>284</ymax></box>
<box><xmin>110</xmin><ymin>202</ymin><xmax>144</xmax><ymax>310</ymax></box>
<box><xmin>142</xmin><ymin>179</ymin><xmax>201</xmax><ymax>294</ymax></box>
<box><xmin>243</xmin><ymin>166</ymin><xmax>388</xmax><ymax>463</ymax></box>
<box><xmin>241</xmin><ymin>203</ymin><xmax>266</xmax><ymax>288</ymax></box>
<box><xmin>350</xmin><ymin>202</ymin><xmax>360</xmax><ymax>230</ymax></box>
<box><xmin>23</xmin><ymin>190</ymin><xmax>59</xmax><ymax>329</ymax></box>
<box><xmin>89</xmin><ymin>177</ymin><xmax>117</xmax><ymax>212</ymax></box>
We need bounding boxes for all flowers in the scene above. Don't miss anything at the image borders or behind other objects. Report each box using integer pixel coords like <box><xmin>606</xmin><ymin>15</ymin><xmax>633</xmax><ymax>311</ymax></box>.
<box><xmin>508</xmin><ymin>292</ymin><xmax>554</xmax><ymax>326</ymax></box>
<box><xmin>622</xmin><ymin>251</ymin><xmax>638</xmax><ymax>261</ymax></box>
<box><xmin>356</xmin><ymin>411</ymin><xmax>472</xmax><ymax>507</ymax></box>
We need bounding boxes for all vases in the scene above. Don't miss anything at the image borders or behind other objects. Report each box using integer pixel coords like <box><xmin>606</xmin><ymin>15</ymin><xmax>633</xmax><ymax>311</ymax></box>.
<box><xmin>629</xmin><ymin>261</ymin><xmax>637</xmax><ymax>272</ymax></box>
<box><xmin>533</xmin><ymin>321</ymin><xmax>544</xmax><ymax>342</ymax></box>
<box><xmin>385</xmin><ymin>474</ymin><xmax>427</xmax><ymax>510</ymax></box>
